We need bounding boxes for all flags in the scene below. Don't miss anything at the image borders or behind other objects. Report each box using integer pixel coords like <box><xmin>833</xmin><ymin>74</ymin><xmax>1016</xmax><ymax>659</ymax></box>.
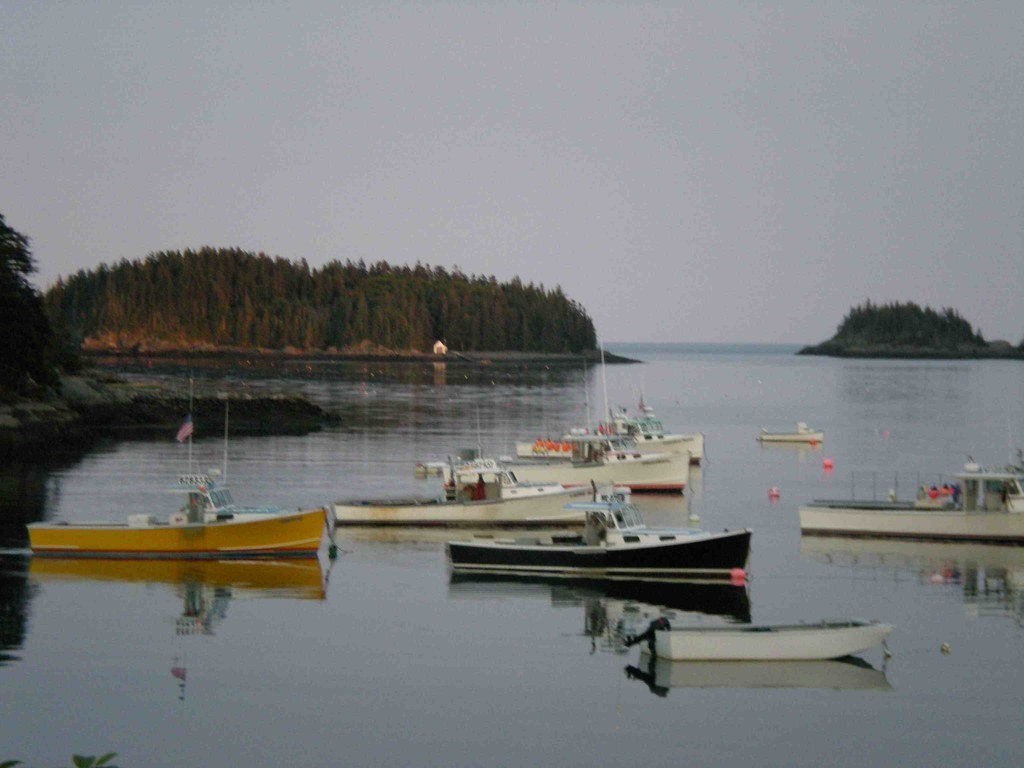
<box><xmin>176</xmin><ymin>415</ymin><xmax>193</xmax><ymax>442</ymax></box>
<box><xmin>639</xmin><ymin>396</ymin><xmax>645</xmax><ymax>408</ymax></box>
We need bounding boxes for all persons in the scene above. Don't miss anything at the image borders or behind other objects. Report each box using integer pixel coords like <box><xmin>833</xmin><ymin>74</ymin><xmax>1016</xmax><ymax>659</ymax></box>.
<box><xmin>929</xmin><ymin>483</ymin><xmax>961</xmax><ymax>504</ymax></box>
<box><xmin>533</xmin><ymin>437</ymin><xmax>579</xmax><ymax>451</ymax></box>
<box><xmin>583</xmin><ymin>511</ymin><xmax>615</xmax><ymax>546</ymax></box>
<box><xmin>1001</xmin><ymin>479</ymin><xmax>1014</xmax><ymax>509</ymax></box>
<box><xmin>624</xmin><ymin>607</ymin><xmax>671</xmax><ymax>648</ymax></box>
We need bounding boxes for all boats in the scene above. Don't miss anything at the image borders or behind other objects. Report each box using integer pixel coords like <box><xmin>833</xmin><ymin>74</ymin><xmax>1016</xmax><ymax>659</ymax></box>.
<box><xmin>26</xmin><ymin>553</ymin><xmax>328</xmax><ymax>603</ymax></box>
<box><xmin>445</xmin><ymin>569</ymin><xmax>752</xmax><ymax>626</ymax></box>
<box><xmin>327</xmin><ymin>448</ymin><xmax>632</xmax><ymax>527</ymax></box>
<box><xmin>24</xmin><ymin>370</ymin><xmax>338</xmax><ymax>557</ymax></box>
<box><xmin>797</xmin><ymin>455</ymin><xmax>1023</xmax><ymax>537</ymax></box>
<box><xmin>623</xmin><ymin>649</ymin><xmax>896</xmax><ymax>699</ymax></box>
<box><xmin>621</xmin><ymin>617</ymin><xmax>898</xmax><ymax>660</ymax></box>
<box><xmin>493</xmin><ymin>354</ymin><xmax>692</xmax><ymax>493</ymax></box>
<box><xmin>760</xmin><ymin>421</ymin><xmax>826</xmax><ymax>442</ymax></box>
<box><xmin>441</xmin><ymin>487</ymin><xmax>759</xmax><ymax>596</ymax></box>
<box><xmin>334</xmin><ymin>526</ymin><xmax>590</xmax><ymax>549</ymax></box>
<box><xmin>799</xmin><ymin>532</ymin><xmax>1024</xmax><ymax>572</ymax></box>
<box><xmin>515</xmin><ymin>341</ymin><xmax>707</xmax><ymax>465</ymax></box>
